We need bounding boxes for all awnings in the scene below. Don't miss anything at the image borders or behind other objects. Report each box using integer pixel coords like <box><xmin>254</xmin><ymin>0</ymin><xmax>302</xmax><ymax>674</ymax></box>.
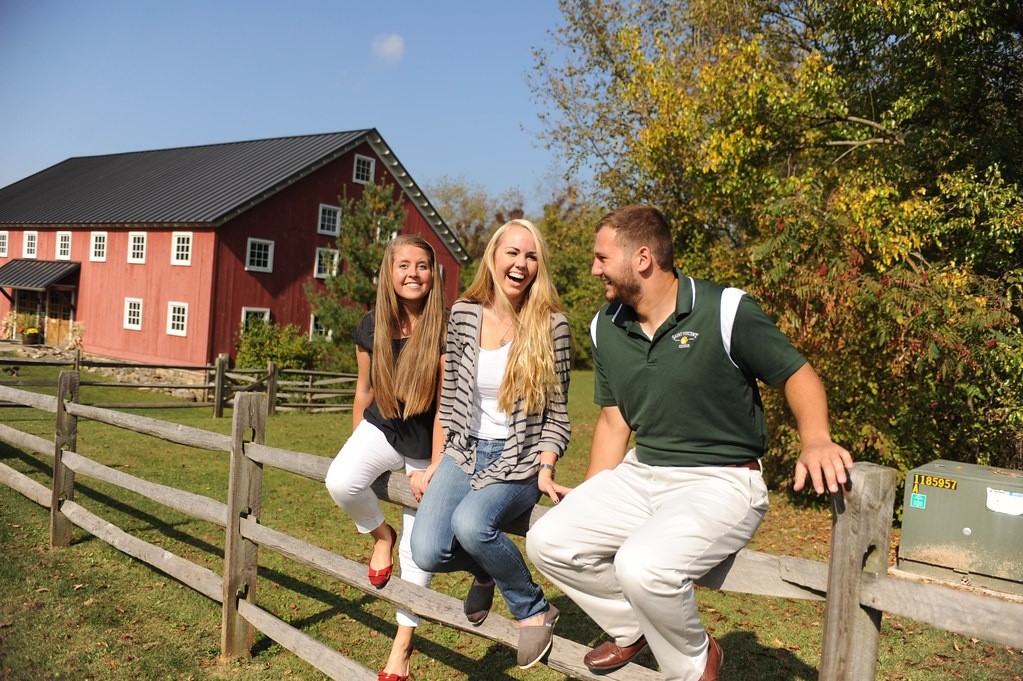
<box><xmin>0</xmin><ymin>258</ymin><xmax>81</xmax><ymax>312</ymax></box>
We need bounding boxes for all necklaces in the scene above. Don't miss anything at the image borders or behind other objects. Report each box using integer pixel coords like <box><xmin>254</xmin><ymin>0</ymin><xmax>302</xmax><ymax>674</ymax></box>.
<box><xmin>491</xmin><ymin>305</ymin><xmax>516</xmax><ymax>347</ymax></box>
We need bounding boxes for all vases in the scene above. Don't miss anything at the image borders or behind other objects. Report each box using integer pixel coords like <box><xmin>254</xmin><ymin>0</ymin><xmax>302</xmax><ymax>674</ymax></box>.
<box><xmin>23</xmin><ymin>333</ymin><xmax>38</xmax><ymax>345</ymax></box>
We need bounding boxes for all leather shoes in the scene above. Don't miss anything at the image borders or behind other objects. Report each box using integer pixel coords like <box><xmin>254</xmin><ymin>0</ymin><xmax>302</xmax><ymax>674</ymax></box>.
<box><xmin>584</xmin><ymin>633</ymin><xmax>649</xmax><ymax>669</ymax></box>
<box><xmin>697</xmin><ymin>632</ymin><xmax>724</xmax><ymax>681</ymax></box>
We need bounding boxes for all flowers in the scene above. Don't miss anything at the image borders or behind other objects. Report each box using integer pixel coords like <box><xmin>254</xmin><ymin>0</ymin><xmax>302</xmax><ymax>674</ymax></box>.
<box><xmin>17</xmin><ymin>324</ymin><xmax>40</xmax><ymax>335</ymax></box>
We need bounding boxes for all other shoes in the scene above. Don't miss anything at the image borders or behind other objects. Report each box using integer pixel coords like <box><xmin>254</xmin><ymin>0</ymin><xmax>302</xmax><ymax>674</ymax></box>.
<box><xmin>516</xmin><ymin>602</ymin><xmax>560</xmax><ymax>668</ymax></box>
<box><xmin>368</xmin><ymin>524</ymin><xmax>396</xmax><ymax>587</ymax></box>
<box><xmin>378</xmin><ymin>657</ymin><xmax>411</xmax><ymax>681</ymax></box>
<box><xmin>466</xmin><ymin>575</ymin><xmax>496</xmax><ymax>624</ymax></box>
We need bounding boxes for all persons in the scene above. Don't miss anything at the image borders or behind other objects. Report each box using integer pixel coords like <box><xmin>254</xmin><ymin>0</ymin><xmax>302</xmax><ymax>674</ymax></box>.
<box><xmin>410</xmin><ymin>218</ymin><xmax>572</xmax><ymax>670</ymax></box>
<box><xmin>325</xmin><ymin>235</ymin><xmax>447</xmax><ymax>681</ymax></box>
<box><xmin>525</xmin><ymin>203</ymin><xmax>853</xmax><ymax>681</ymax></box>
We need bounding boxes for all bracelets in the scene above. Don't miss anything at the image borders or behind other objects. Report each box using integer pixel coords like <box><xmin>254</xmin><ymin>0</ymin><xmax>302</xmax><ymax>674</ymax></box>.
<box><xmin>537</xmin><ymin>464</ymin><xmax>555</xmax><ymax>479</ymax></box>
